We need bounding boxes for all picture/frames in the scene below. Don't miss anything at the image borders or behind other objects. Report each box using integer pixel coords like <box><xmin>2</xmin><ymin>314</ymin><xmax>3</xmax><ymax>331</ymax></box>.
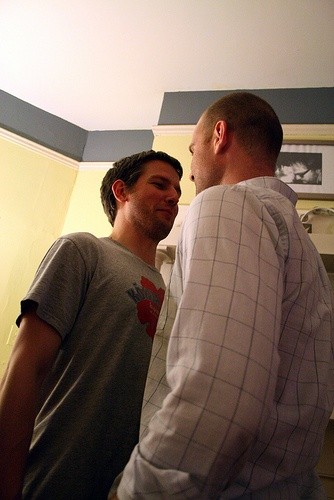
<box><xmin>274</xmin><ymin>139</ymin><xmax>334</xmax><ymax>201</ymax></box>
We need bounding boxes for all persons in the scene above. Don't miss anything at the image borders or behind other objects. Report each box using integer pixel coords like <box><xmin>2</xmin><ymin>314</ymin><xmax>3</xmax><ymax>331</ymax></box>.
<box><xmin>274</xmin><ymin>161</ymin><xmax>320</xmax><ymax>184</ymax></box>
<box><xmin>106</xmin><ymin>88</ymin><xmax>334</xmax><ymax>499</ymax></box>
<box><xmin>0</xmin><ymin>148</ymin><xmax>185</xmax><ymax>500</ymax></box>
